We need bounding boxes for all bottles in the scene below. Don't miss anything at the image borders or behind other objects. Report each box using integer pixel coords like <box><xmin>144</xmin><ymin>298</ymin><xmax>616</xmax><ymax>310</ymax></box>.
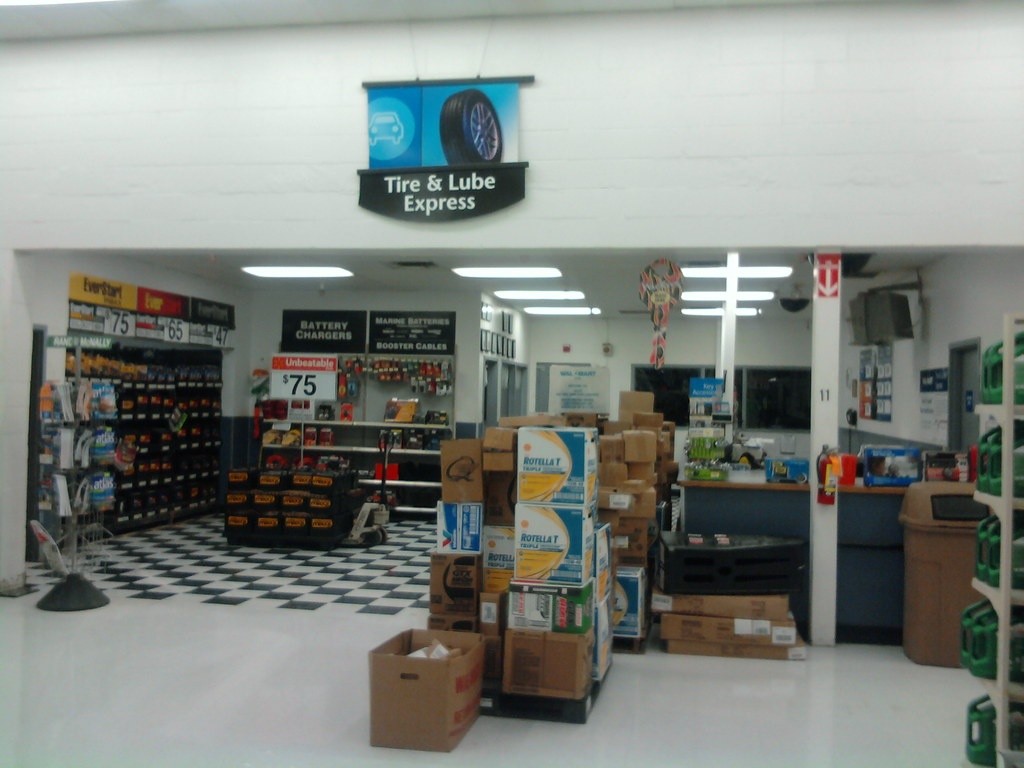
<box><xmin>955</xmin><ymin>457</ymin><xmax>969</xmax><ymax>482</ymax></box>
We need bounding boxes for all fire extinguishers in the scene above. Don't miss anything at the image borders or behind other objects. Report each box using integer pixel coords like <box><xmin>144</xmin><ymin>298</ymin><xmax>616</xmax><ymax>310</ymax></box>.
<box><xmin>816</xmin><ymin>443</ymin><xmax>840</xmax><ymax>505</ymax></box>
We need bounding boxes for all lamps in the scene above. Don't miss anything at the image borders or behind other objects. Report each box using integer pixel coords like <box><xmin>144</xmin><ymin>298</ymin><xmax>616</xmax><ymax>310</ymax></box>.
<box><xmin>778</xmin><ymin>284</ymin><xmax>809</xmax><ymax>312</ymax></box>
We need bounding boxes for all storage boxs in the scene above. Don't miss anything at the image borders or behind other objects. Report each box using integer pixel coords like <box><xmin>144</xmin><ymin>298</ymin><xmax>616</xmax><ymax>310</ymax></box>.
<box><xmin>479</xmin><ymin>589</ymin><xmax>506</xmax><ymax>634</ymax></box>
<box><xmin>923</xmin><ymin>449</ymin><xmax>970</xmax><ymax>483</ymax></box>
<box><xmin>654</xmin><ymin>558</ymin><xmax>797</xmax><ymax>593</ymax></box>
<box><xmin>658</xmin><ymin>529</ymin><xmax>806</xmax><ymax>569</ymax></box>
<box><xmin>483</xmin><ymin>426</ymin><xmax>518</xmax><ymax>451</ymax></box>
<box><xmin>483</xmin><ymin>471</ymin><xmax>518</xmax><ymax>526</ymax></box>
<box><xmin>437</xmin><ymin>500</ymin><xmax>483</xmax><ymax>554</ymax></box>
<box><xmin>368</xmin><ymin>628</ymin><xmax>486</xmax><ymax>754</ymax></box>
<box><xmin>484</xmin><ymin>526</ymin><xmax>516</xmax><ymax>570</ymax></box>
<box><xmin>660</xmin><ymin>611</ymin><xmax>797</xmax><ymax>648</ymax></box>
<box><xmin>485</xmin><ymin>566</ymin><xmax>515</xmax><ymax>593</ymax></box>
<box><xmin>649</xmin><ymin>585</ymin><xmax>790</xmax><ymax>621</ymax></box>
<box><xmin>427</xmin><ymin>613</ymin><xmax>478</xmax><ymax>634</ymax></box>
<box><xmin>502</xmin><ymin>389</ymin><xmax>680</xmax><ymax>727</ymax></box>
<box><xmin>431</xmin><ymin>551</ymin><xmax>484</xmax><ymax>614</ymax></box>
<box><xmin>667</xmin><ymin>637</ymin><xmax>806</xmax><ymax>659</ymax></box>
<box><xmin>440</xmin><ymin>438</ymin><xmax>484</xmax><ymax>503</ymax></box>
<box><xmin>483</xmin><ymin>451</ymin><xmax>515</xmax><ymax>471</ymax></box>
<box><xmin>863</xmin><ymin>448</ymin><xmax>921</xmax><ymax>486</ymax></box>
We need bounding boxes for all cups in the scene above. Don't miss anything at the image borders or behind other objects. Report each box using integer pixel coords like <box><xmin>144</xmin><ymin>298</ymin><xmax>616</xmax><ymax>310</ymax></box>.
<box><xmin>838</xmin><ymin>455</ymin><xmax>857</xmax><ymax>486</ymax></box>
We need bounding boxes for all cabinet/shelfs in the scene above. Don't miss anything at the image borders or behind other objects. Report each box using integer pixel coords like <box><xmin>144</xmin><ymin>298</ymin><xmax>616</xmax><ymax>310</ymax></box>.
<box><xmin>64</xmin><ymin>347</ymin><xmax>225</xmax><ymax>545</ymax></box>
<box><xmin>260</xmin><ymin>416</ymin><xmax>457</xmax><ymax>515</ymax></box>
<box><xmin>960</xmin><ymin>312</ymin><xmax>1024</xmax><ymax>768</ymax></box>
<box><xmin>37</xmin><ymin>374</ymin><xmax>116</xmax><ymax>611</ymax></box>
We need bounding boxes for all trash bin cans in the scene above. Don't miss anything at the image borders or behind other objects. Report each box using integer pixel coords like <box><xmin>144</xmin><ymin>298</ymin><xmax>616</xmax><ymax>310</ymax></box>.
<box><xmin>897</xmin><ymin>481</ymin><xmax>989</xmax><ymax>668</ymax></box>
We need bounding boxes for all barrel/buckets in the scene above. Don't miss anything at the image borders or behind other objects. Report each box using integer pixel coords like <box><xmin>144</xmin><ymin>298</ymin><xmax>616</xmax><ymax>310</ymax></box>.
<box><xmin>979</xmin><ymin>331</ymin><xmax>1024</xmax><ymax>405</ymax></box>
<box><xmin>977</xmin><ymin>426</ymin><xmax>1001</xmax><ymax>492</ymax></box>
<box><xmin>969</xmin><ymin>606</ymin><xmax>1024</xmax><ymax>683</ymax></box>
<box><xmin>974</xmin><ymin>511</ymin><xmax>1024</xmax><ymax>589</ymax></box>
<box><xmin>987</xmin><ymin>419</ymin><xmax>1024</xmax><ymax>498</ymax></box>
<box><xmin>959</xmin><ymin>598</ymin><xmax>991</xmax><ymax>670</ymax></box>
<box><xmin>965</xmin><ymin>693</ymin><xmax>1024</xmax><ymax>765</ymax></box>
